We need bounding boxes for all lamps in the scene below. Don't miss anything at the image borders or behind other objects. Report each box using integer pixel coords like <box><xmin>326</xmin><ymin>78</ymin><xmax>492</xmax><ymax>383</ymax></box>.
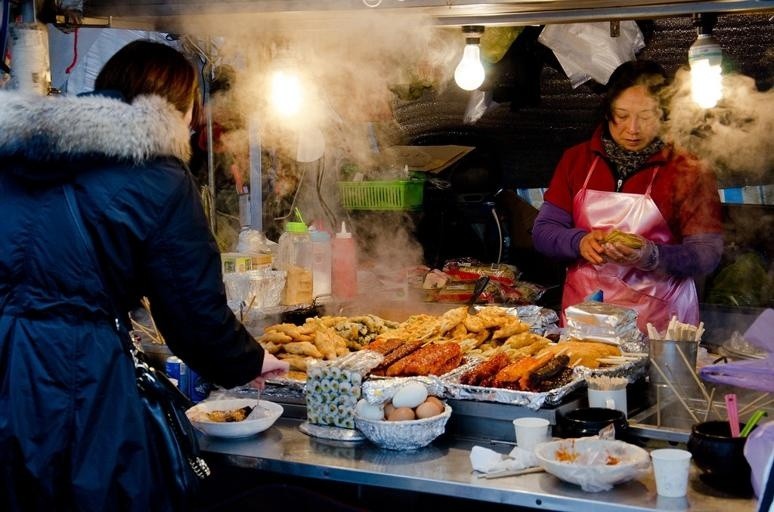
<box><xmin>687</xmin><ymin>12</ymin><xmax>724</xmax><ymax>106</ymax></box>
<box><xmin>454</xmin><ymin>21</ymin><xmax>485</xmax><ymax>91</ymax></box>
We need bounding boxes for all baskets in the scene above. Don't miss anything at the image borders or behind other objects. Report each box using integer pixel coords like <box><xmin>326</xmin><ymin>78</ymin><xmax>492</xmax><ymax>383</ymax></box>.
<box><xmin>338</xmin><ymin>180</ymin><xmax>423</xmax><ymax>212</ymax></box>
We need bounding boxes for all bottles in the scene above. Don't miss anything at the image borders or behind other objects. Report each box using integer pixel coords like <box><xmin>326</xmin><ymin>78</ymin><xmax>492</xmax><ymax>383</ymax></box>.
<box><xmin>277</xmin><ymin>223</ymin><xmax>314</xmax><ymax>309</ymax></box>
<box><xmin>307</xmin><ymin>221</ymin><xmax>332</xmax><ymax>299</ymax></box>
<box><xmin>331</xmin><ymin>221</ymin><xmax>358</xmax><ymax>300</ymax></box>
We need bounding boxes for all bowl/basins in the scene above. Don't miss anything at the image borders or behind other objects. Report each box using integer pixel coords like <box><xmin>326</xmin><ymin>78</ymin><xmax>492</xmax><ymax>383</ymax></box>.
<box><xmin>184</xmin><ymin>397</ymin><xmax>283</xmax><ymax>441</ymax></box>
<box><xmin>535</xmin><ymin>440</ymin><xmax>646</xmax><ymax>493</ymax></box>
<box><xmin>352</xmin><ymin>403</ymin><xmax>453</xmax><ymax>453</ymax></box>
<box><xmin>556</xmin><ymin>407</ymin><xmax>630</xmax><ymax>441</ymax></box>
<box><xmin>683</xmin><ymin>422</ymin><xmax>753</xmax><ymax>478</ymax></box>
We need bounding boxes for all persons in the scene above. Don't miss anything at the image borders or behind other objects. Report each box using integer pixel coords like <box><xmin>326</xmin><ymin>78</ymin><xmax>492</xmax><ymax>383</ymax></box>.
<box><xmin>529</xmin><ymin>58</ymin><xmax>727</xmax><ymax>342</ymax></box>
<box><xmin>1</xmin><ymin>39</ymin><xmax>288</xmax><ymax>510</ymax></box>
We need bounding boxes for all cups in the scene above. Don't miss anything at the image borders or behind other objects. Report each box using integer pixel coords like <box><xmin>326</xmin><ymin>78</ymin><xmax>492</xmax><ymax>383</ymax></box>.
<box><xmin>512</xmin><ymin>416</ymin><xmax>549</xmax><ymax>452</ymax></box>
<box><xmin>585</xmin><ymin>388</ymin><xmax>628</xmax><ymax>420</ymax></box>
<box><xmin>655</xmin><ymin>495</ymin><xmax>688</xmax><ymax>511</ymax></box>
<box><xmin>647</xmin><ymin>337</ymin><xmax>698</xmax><ymax>403</ymax></box>
<box><xmin>648</xmin><ymin>448</ymin><xmax>692</xmax><ymax>498</ymax></box>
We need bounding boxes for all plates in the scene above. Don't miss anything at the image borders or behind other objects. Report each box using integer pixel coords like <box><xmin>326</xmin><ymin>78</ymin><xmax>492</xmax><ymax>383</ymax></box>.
<box><xmin>721</xmin><ymin>335</ymin><xmax>765</xmax><ymax>362</ymax></box>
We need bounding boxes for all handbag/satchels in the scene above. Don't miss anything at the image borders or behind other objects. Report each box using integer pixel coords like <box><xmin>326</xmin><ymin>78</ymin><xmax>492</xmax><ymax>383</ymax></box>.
<box><xmin>131</xmin><ymin>351</ymin><xmax>211</xmax><ymax>488</ymax></box>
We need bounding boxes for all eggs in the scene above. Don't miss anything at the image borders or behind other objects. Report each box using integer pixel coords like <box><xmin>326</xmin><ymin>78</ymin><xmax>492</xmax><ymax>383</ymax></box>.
<box><xmin>356</xmin><ymin>382</ymin><xmax>444</xmax><ymax>422</ymax></box>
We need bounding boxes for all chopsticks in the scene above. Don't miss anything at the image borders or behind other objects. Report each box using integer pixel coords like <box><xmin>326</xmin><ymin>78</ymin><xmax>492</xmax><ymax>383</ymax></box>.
<box><xmin>584</xmin><ymin>374</ymin><xmax>630</xmax><ymax>391</ymax></box>
<box><xmin>644</xmin><ymin>314</ymin><xmax>706</xmax><ymax>342</ymax></box>
<box><xmin>476</xmin><ymin>465</ymin><xmax>545</xmax><ymax>480</ymax></box>
